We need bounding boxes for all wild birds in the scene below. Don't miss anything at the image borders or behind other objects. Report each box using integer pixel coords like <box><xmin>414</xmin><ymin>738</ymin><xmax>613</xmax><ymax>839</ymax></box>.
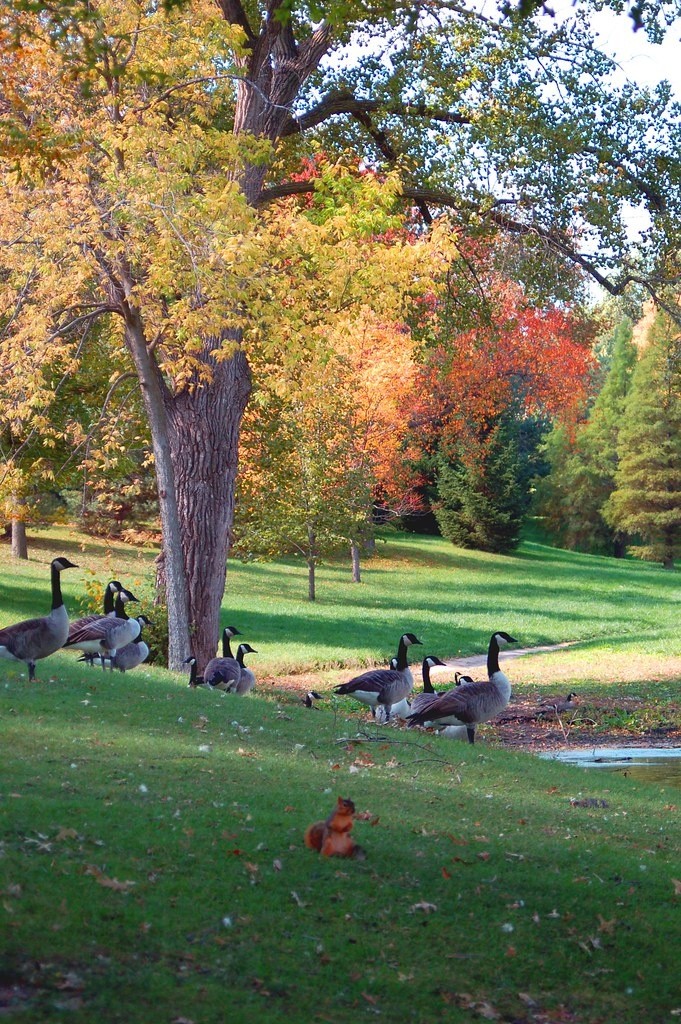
<box><xmin>334</xmin><ymin>631</ymin><xmax>521</xmax><ymax>744</ymax></box>
<box><xmin>181</xmin><ymin>626</ymin><xmax>258</xmax><ymax>695</ymax></box>
<box><xmin>540</xmin><ymin>693</ymin><xmax>578</xmax><ymax>710</ymax></box>
<box><xmin>306</xmin><ymin>691</ymin><xmax>325</xmax><ymax>709</ymax></box>
<box><xmin>0</xmin><ymin>557</ymin><xmax>155</xmax><ymax>681</ymax></box>
